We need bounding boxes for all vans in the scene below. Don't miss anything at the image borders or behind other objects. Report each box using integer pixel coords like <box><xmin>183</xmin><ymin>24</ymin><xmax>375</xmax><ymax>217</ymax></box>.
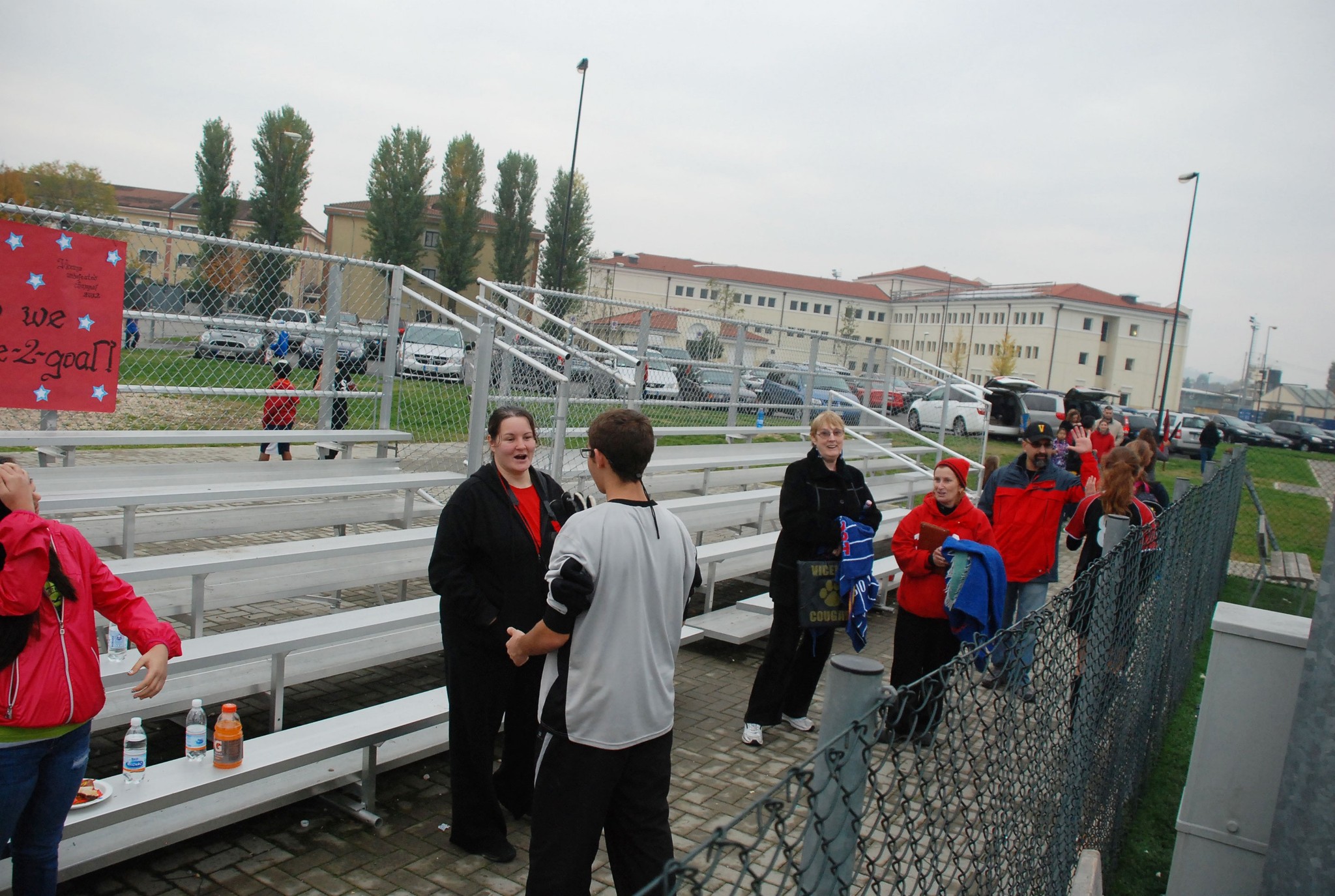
<box><xmin>588</xmin><ymin>345</ymin><xmax>680</xmax><ymax>401</ymax></box>
<box><xmin>647</xmin><ymin>345</ymin><xmax>694</xmax><ymax>384</ymax></box>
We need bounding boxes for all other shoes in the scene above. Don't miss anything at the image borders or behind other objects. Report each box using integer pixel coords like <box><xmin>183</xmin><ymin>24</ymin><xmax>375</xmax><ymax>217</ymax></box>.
<box><xmin>482</xmin><ymin>841</ymin><xmax>516</xmax><ymax>863</ymax></box>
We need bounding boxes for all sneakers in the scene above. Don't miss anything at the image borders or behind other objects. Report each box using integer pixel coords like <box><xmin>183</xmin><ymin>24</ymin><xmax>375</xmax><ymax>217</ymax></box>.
<box><xmin>741</xmin><ymin>723</ymin><xmax>764</xmax><ymax>746</ymax></box>
<box><xmin>911</xmin><ymin>732</ymin><xmax>932</xmax><ymax>747</ymax></box>
<box><xmin>1009</xmin><ymin>681</ymin><xmax>1036</xmax><ymax>702</ymax></box>
<box><xmin>874</xmin><ymin>728</ymin><xmax>911</xmax><ymax>744</ymax></box>
<box><xmin>982</xmin><ymin>668</ymin><xmax>1007</xmax><ymax>688</ymax></box>
<box><xmin>782</xmin><ymin>713</ymin><xmax>815</xmax><ymax>731</ymax></box>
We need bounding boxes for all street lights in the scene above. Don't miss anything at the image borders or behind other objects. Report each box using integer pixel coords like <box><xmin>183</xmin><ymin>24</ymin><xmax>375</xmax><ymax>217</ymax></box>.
<box><xmin>346</xmin><ymin>211</ymin><xmax>355</xmax><ymax>313</ymax></box>
<box><xmin>936</xmin><ymin>275</ymin><xmax>958</xmax><ymax>386</ymax></box>
<box><xmin>559</xmin><ymin>56</ymin><xmax>590</xmax><ymax>289</ymax></box>
<box><xmin>1155</xmin><ymin>168</ymin><xmax>1201</xmax><ymax>431</ymax></box>
<box><xmin>1256</xmin><ymin>325</ymin><xmax>1278</xmax><ymax>424</ymax></box>
<box><xmin>263</xmin><ymin>130</ymin><xmax>303</xmax><ymax>318</ymax></box>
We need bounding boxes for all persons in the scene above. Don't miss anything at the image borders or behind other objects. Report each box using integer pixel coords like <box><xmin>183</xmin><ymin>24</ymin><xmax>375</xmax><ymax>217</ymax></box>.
<box><xmin>742</xmin><ymin>411</ymin><xmax>883</xmax><ymax>744</ymax></box>
<box><xmin>428</xmin><ymin>407</ymin><xmax>565</xmax><ymax>863</ymax></box>
<box><xmin>263</xmin><ymin>322</ymin><xmax>289</xmax><ymax>365</ymax></box>
<box><xmin>313</xmin><ymin>352</ymin><xmax>351</xmax><ymax>459</ymax></box>
<box><xmin>506</xmin><ymin>409</ymin><xmax>701</xmax><ymax>895</ymax></box>
<box><xmin>977</xmin><ymin>422</ymin><xmax>1100</xmax><ymax>702</ymax></box>
<box><xmin>1051</xmin><ymin>406</ymin><xmax>1124</xmax><ymax>476</ymax></box>
<box><xmin>1125</xmin><ymin>428</ymin><xmax>1171</xmax><ymax>594</ymax></box>
<box><xmin>259</xmin><ymin>360</ymin><xmax>300</xmax><ymax>461</ymax></box>
<box><xmin>0</xmin><ymin>456</ymin><xmax>183</xmax><ymax>896</ymax></box>
<box><xmin>123</xmin><ymin>304</ymin><xmax>140</xmax><ymax>349</ymax></box>
<box><xmin>1064</xmin><ymin>447</ymin><xmax>1161</xmax><ymax>735</ymax></box>
<box><xmin>879</xmin><ymin>458</ymin><xmax>1003</xmax><ymax>744</ymax></box>
<box><xmin>1199</xmin><ymin>421</ymin><xmax>1220</xmax><ymax>474</ymax></box>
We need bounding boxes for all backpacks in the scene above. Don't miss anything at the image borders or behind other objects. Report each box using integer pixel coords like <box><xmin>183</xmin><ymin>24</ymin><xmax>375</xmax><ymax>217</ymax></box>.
<box><xmin>1134</xmin><ymin>480</ymin><xmax>1165</xmax><ymax>518</ymax></box>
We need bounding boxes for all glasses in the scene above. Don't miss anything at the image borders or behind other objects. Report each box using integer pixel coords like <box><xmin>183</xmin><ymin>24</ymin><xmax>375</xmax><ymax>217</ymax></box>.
<box><xmin>1024</xmin><ymin>439</ymin><xmax>1053</xmax><ymax>449</ymax></box>
<box><xmin>815</xmin><ymin>429</ymin><xmax>844</xmax><ymax>439</ymax></box>
<box><xmin>580</xmin><ymin>447</ymin><xmax>614</xmax><ymax>466</ymax></box>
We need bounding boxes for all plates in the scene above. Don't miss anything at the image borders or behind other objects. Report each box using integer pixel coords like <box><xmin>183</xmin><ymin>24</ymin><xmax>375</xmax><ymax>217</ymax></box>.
<box><xmin>70</xmin><ymin>778</ymin><xmax>113</xmax><ymax>809</ymax></box>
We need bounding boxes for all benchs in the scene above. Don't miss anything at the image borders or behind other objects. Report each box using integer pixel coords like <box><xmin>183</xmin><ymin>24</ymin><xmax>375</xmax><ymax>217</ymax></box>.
<box><xmin>0</xmin><ymin>427</ymin><xmax>976</xmax><ymax>896</ymax></box>
<box><xmin>1248</xmin><ymin>515</ymin><xmax>1315</xmax><ymax>616</ymax></box>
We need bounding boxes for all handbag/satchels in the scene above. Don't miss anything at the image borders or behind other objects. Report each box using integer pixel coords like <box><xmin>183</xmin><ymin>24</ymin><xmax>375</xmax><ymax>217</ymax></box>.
<box><xmin>797</xmin><ymin>557</ymin><xmax>849</xmax><ymax>630</ymax></box>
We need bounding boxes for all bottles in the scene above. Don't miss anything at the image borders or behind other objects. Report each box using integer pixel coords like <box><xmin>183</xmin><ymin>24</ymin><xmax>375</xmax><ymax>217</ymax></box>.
<box><xmin>757</xmin><ymin>408</ymin><xmax>764</xmax><ymax>428</ymax></box>
<box><xmin>107</xmin><ymin>621</ymin><xmax>128</xmax><ymax>663</ymax></box>
<box><xmin>213</xmin><ymin>704</ymin><xmax>243</xmax><ymax>769</ymax></box>
<box><xmin>185</xmin><ymin>699</ymin><xmax>207</xmax><ymax>762</ymax></box>
<box><xmin>122</xmin><ymin>717</ymin><xmax>147</xmax><ymax>785</ymax></box>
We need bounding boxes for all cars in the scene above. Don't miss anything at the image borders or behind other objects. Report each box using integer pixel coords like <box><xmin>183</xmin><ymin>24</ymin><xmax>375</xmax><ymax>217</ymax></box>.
<box><xmin>266</xmin><ymin>307</ymin><xmax>407</xmax><ymax>361</ymax></box>
<box><xmin>195</xmin><ymin>312</ymin><xmax>270</xmax><ymax>364</ymax></box>
<box><xmin>678</xmin><ymin>368</ymin><xmax>759</xmax><ymax>416</ymax></box>
<box><xmin>763</xmin><ymin>364</ymin><xmax>862</xmax><ymax>428</ymax></box>
<box><xmin>1017</xmin><ymin>384</ymin><xmax>1335</xmax><ymax>459</ymax></box>
<box><xmin>394</xmin><ymin>322</ymin><xmax>472</xmax><ymax>383</ymax></box>
<box><xmin>297</xmin><ymin>321</ymin><xmax>373</xmax><ymax>375</ymax></box>
<box><xmin>739</xmin><ymin>358</ymin><xmax>938</xmax><ymax>416</ymax></box>
<box><xmin>490</xmin><ymin>332</ymin><xmax>592</xmax><ymax>396</ymax></box>
<box><xmin>906</xmin><ymin>375</ymin><xmax>1041</xmax><ymax>438</ymax></box>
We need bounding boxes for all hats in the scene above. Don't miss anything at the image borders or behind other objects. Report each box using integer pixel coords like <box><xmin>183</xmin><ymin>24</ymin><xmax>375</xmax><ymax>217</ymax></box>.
<box><xmin>1025</xmin><ymin>421</ymin><xmax>1053</xmax><ymax>442</ymax></box>
<box><xmin>273</xmin><ymin>359</ymin><xmax>291</xmax><ymax>377</ymax></box>
<box><xmin>933</xmin><ymin>458</ymin><xmax>970</xmax><ymax>489</ymax></box>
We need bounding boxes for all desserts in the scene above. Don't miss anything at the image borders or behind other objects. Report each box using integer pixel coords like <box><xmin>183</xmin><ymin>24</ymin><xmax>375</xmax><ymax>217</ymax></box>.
<box><xmin>76</xmin><ymin>786</ymin><xmax>99</xmax><ymax>802</ymax></box>
